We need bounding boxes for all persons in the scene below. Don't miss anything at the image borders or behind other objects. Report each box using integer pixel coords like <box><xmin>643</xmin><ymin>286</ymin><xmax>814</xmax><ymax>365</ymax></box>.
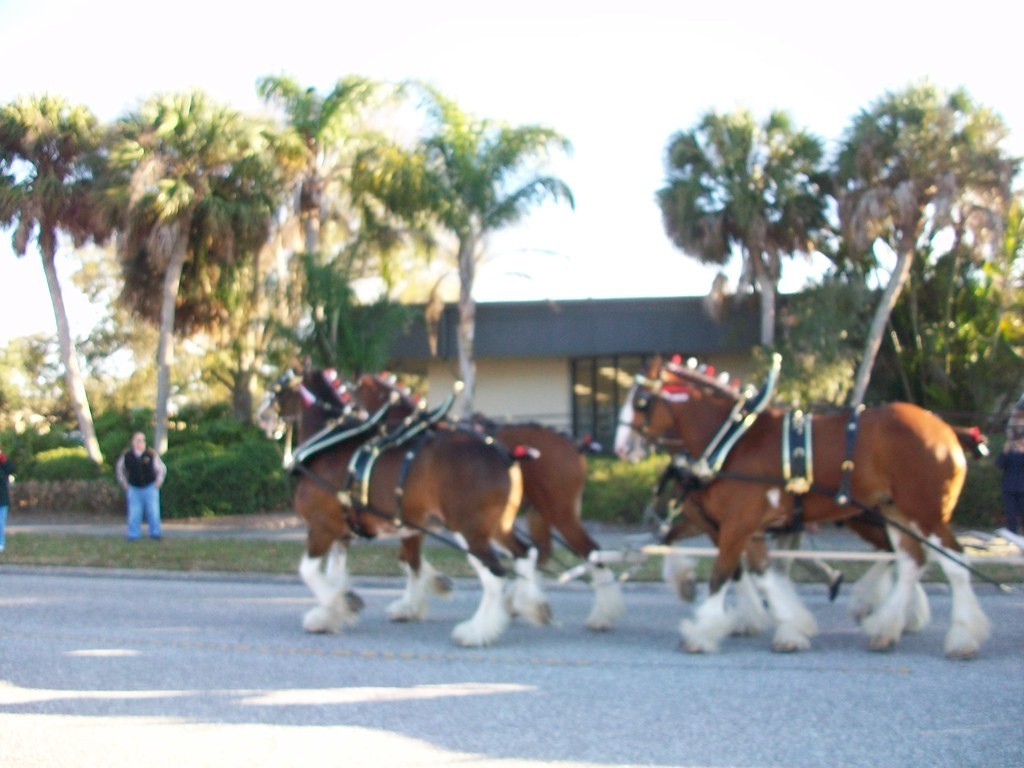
<box><xmin>995</xmin><ymin>434</ymin><xmax>1024</xmax><ymax>534</ymax></box>
<box><xmin>0</xmin><ymin>447</ymin><xmax>18</xmax><ymax>554</ymax></box>
<box><xmin>116</xmin><ymin>432</ymin><xmax>167</xmax><ymax>541</ymax></box>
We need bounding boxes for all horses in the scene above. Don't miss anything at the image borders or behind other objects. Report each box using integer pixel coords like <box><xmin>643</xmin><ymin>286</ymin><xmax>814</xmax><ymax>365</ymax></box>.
<box><xmin>257</xmin><ymin>366</ymin><xmax>624</xmax><ymax>647</ymax></box>
<box><xmin>613</xmin><ymin>359</ymin><xmax>991</xmax><ymax>659</ymax></box>
